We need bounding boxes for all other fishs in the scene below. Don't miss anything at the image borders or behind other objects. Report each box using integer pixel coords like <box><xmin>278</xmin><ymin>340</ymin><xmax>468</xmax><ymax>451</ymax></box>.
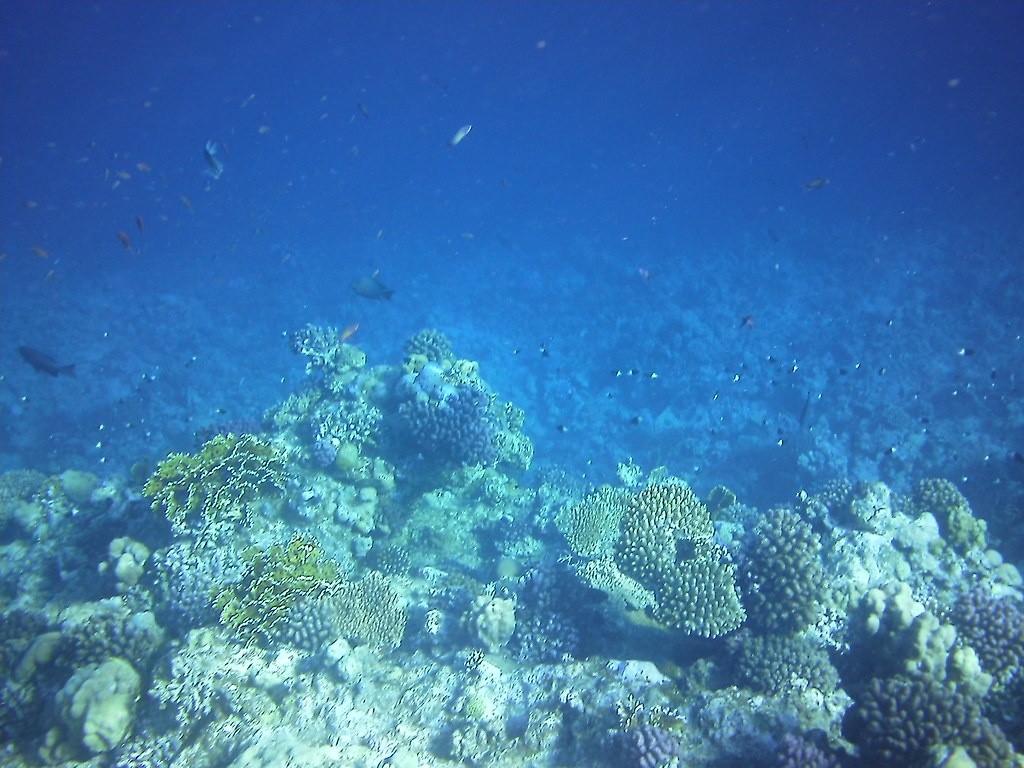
<box><xmin>1</xmin><ymin>37</ymin><xmax>1024</xmax><ymax>511</ymax></box>
